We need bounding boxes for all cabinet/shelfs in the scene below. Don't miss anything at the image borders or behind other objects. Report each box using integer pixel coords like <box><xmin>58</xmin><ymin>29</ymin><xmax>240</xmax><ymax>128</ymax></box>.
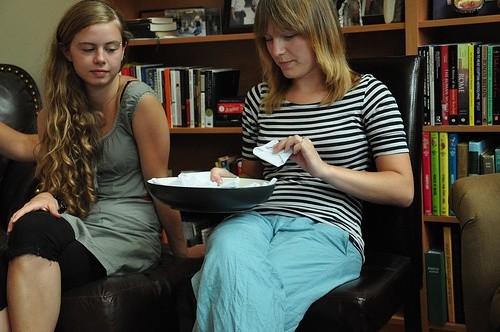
<box><xmin>99</xmin><ymin>0</ymin><xmax>500</xmax><ymax>332</ymax></box>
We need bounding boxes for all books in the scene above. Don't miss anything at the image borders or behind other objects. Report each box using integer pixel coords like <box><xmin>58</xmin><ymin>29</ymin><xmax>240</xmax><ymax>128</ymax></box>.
<box><xmin>122</xmin><ymin>16</ymin><xmax>183</xmax><ymax>41</ymax></box>
<box><xmin>425</xmin><ymin>223</ymin><xmax>462</xmax><ymax>327</ymax></box>
<box><xmin>121</xmin><ymin>64</ymin><xmax>240</xmax><ymax>130</ymax></box>
<box><xmin>417</xmin><ymin>44</ymin><xmax>500</xmax><ymax>126</ymax></box>
<box><xmin>421</xmin><ymin>132</ymin><xmax>500</xmax><ymax>216</ymax></box>
<box><xmin>215</xmin><ymin>153</ymin><xmax>242</xmax><ymax>175</ymax></box>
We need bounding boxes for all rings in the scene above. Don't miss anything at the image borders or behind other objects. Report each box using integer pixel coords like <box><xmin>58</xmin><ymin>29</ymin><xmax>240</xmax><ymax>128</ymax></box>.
<box><xmin>301</xmin><ymin>136</ymin><xmax>304</xmax><ymax>143</ymax></box>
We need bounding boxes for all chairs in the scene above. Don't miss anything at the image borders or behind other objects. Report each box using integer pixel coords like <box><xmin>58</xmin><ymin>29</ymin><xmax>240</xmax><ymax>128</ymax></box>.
<box><xmin>0</xmin><ymin>64</ymin><xmax>201</xmax><ymax>332</ymax></box>
<box><xmin>297</xmin><ymin>57</ymin><xmax>425</xmax><ymax>332</ymax></box>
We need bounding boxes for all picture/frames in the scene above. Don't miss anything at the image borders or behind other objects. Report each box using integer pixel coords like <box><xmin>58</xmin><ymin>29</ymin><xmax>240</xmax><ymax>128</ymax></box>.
<box><xmin>163</xmin><ymin>8</ymin><xmax>208</xmax><ymax>36</ymax></box>
<box><xmin>222</xmin><ymin>0</ymin><xmax>256</xmax><ymax>36</ymax></box>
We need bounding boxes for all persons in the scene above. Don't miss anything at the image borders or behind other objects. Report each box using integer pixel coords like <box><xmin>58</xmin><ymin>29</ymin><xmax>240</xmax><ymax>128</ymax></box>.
<box><xmin>0</xmin><ymin>121</ymin><xmax>40</xmax><ymax>163</ymax></box>
<box><xmin>192</xmin><ymin>0</ymin><xmax>414</xmax><ymax>332</ymax></box>
<box><xmin>0</xmin><ymin>0</ymin><xmax>207</xmax><ymax>332</ymax></box>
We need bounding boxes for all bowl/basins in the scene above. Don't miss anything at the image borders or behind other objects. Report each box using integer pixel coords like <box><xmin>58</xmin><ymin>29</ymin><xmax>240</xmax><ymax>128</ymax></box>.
<box><xmin>145</xmin><ymin>176</ymin><xmax>277</xmax><ymax>213</ymax></box>
